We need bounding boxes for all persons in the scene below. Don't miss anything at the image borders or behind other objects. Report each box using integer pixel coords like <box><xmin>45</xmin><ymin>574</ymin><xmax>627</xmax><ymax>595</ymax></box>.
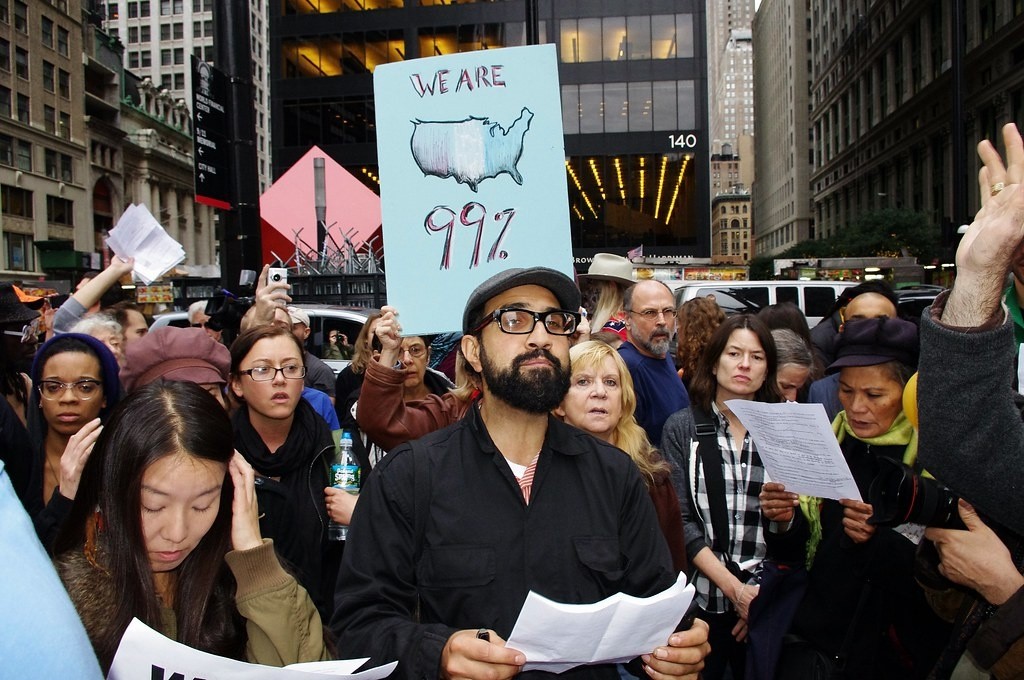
<box><xmin>916</xmin><ymin>121</ymin><xmax>1024</xmax><ymax>679</ymax></box>
<box><xmin>328</xmin><ymin>266</ymin><xmax>712</xmax><ymax>680</ymax></box>
<box><xmin>580</xmin><ymin>245</ymin><xmax>1024</xmax><ymax>680</ymax></box>
<box><xmin>0</xmin><ymin>254</ymin><xmax>483</xmax><ymax>680</ymax></box>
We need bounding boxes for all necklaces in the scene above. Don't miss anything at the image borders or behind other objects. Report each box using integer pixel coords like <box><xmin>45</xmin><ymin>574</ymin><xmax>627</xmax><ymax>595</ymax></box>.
<box><xmin>46</xmin><ymin>452</ymin><xmax>61</xmax><ymax>487</ymax></box>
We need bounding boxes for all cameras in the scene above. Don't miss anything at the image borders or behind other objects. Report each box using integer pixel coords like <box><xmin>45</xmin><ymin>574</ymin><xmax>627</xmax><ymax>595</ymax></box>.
<box><xmin>865</xmin><ymin>453</ymin><xmax>1024</xmax><ymax>570</ymax></box>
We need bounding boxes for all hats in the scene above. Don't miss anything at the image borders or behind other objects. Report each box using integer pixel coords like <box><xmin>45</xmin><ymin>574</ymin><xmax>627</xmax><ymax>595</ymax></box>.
<box><xmin>288</xmin><ymin>307</ymin><xmax>311</xmax><ymax>328</ymax></box>
<box><xmin>577</xmin><ymin>253</ymin><xmax>638</xmax><ymax>284</ymax></box>
<box><xmin>462</xmin><ymin>266</ymin><xmax>581</xmax><ymax>333</ymax></box>
<box><xmin>823</xmin><ymin>315</ymin><xmax>918</xmax><ymax>375</ymax></box>
<box><xmin>119</xmin><ymin>325</ymin><xmax>231</xmax><ymax>395</ymax></box>
<box><xmin>0</xmin><ymin>282</ymin><xmax>44</xmax><ymax>323</ymax></box>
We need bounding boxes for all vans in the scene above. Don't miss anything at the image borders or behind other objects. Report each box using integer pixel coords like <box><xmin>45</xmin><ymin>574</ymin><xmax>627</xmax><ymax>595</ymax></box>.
<box><xmin>670</xmin><ymin>278</ymin><xmax>865</xmax><ymax>330</ymax></box>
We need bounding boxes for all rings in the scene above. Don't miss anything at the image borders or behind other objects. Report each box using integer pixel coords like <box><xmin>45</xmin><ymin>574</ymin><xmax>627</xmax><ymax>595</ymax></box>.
<box><xmin>991</xmin><ymin>183</ymin><xmax>1004</xmax><ymax>196</ymax></box>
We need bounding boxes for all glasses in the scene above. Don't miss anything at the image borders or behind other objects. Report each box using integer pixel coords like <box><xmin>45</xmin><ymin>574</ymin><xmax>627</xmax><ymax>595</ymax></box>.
<box><xmin>36</xmin><ymin>379</ymin><xmax>100</xmax><ymax>400</ymax></box>
<box><xmin>399</xmin><ymin>345</ymin><xmax>428</xmax><ymax>359</ymax></box>
<box><xmin>236</xmin><ymin>365</ymin><xmax>307</xmax><ymax>381</ymax></box>
<box><xmin>629</xmin><ymin>309</ymin><xmax>678</xmax><ymax>322</ymax></box>
<box><xmin>469</xmin><ymin>308</ymin><xmax>580</xmax><ymax>337</ymax></box>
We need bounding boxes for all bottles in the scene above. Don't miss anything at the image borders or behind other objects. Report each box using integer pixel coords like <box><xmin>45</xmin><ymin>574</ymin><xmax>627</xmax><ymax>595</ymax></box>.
<box><xmin>327</xmin><ymin>432</ymin><xmax>362</xmax><ymax>542</ymax></box>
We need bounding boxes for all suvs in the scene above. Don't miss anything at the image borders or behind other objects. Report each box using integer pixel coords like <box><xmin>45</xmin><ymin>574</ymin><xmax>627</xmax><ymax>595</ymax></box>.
<box><xmin>892</xmin><ymin>283</ymin><xmax>950</xmax><ymax>317</ymax></box>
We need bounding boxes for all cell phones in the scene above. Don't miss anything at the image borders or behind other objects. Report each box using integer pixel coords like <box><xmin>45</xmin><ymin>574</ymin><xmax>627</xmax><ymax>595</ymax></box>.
<box><xmin>268</xmin><ymin>268</ymin><xmax>288</xmax><ymax>305</ymax></box>
